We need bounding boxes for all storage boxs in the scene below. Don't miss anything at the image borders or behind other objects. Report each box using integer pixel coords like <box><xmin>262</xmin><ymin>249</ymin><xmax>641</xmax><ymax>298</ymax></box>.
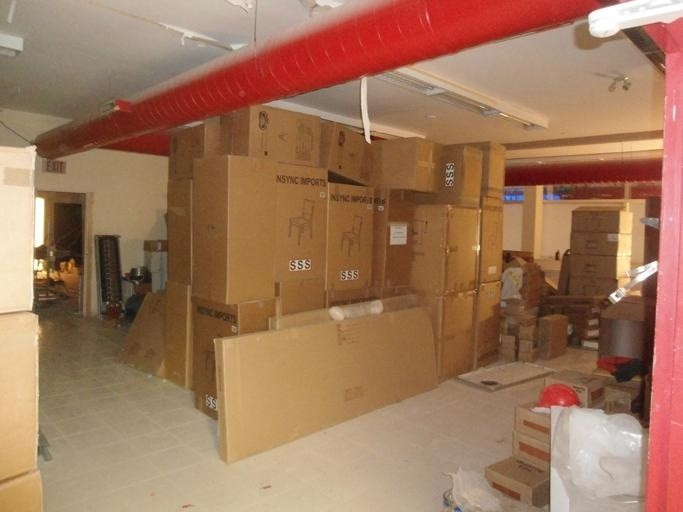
<box><xmin>119</xmin><ymin>106</ymin><xmax>513</xmax><ymax>459</ymax></box>
<box><xmin>1</xmin><ymin>143</ymin><xmax>46</xmax><ymax>510</ymax></box>
<box><xmin>497</xmin><ymin>201</ymin><xmax>644</xmax><ymax>363</ymax></box>
<box><xmin>485</xmin><ymin>366</ymin><xmax>651</xmax><ymax>512</ymax></box>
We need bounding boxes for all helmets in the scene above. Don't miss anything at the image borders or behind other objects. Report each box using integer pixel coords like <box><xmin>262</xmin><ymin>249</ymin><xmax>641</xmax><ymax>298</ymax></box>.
<box><xmin>538</xmin><ymin>385</ymin><xmax>579</xmax><ymax>410</ymax></box>
